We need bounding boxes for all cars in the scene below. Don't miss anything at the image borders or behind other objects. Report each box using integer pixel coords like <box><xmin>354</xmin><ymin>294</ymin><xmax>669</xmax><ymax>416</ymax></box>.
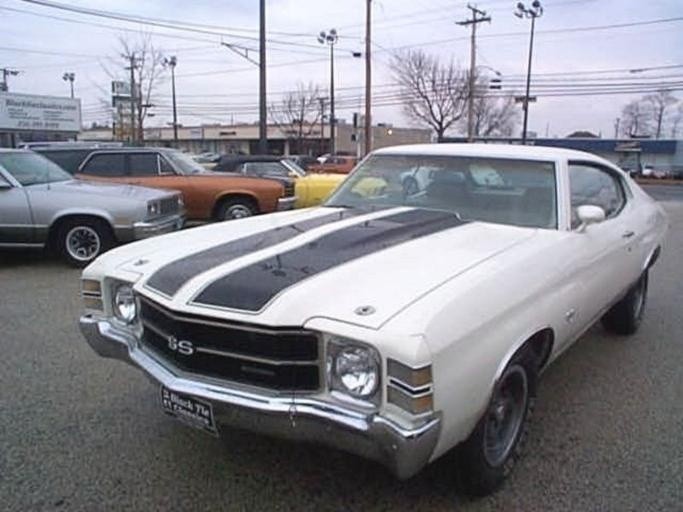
<box><xmin>77</xmin><ymin>136</ymin><xmax>669</xmax><ymax>491</ymax></box>
<box><xmin>0</xmin><ymin>146</ymin><xmax>184</xmax><ymax>267</ymax></box>
<box><xmin>18</xmin><ymin>140</ymin><xmax>504</xmax><ymax>224</ymax></box>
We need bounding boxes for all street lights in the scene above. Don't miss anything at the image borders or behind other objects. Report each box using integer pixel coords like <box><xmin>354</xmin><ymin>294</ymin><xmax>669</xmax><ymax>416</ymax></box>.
<box><xmin>159</xmin><ymin>54</ymin><xmax>178</xmax><ymax>150</ymax></box>
<box><xmin>507</xmin><ymin>0</ymin><xmax>547</xmax><ymax>145</ymax></box>
<box><xmin>61</xmin><ymin>71</ymin><xmax>75</xmax><ymax>98</ymax></box>
<box><xmin>316</xmin><ymin>27</ymin><xmax>341</xmax><ymax>157</ymax></box>
